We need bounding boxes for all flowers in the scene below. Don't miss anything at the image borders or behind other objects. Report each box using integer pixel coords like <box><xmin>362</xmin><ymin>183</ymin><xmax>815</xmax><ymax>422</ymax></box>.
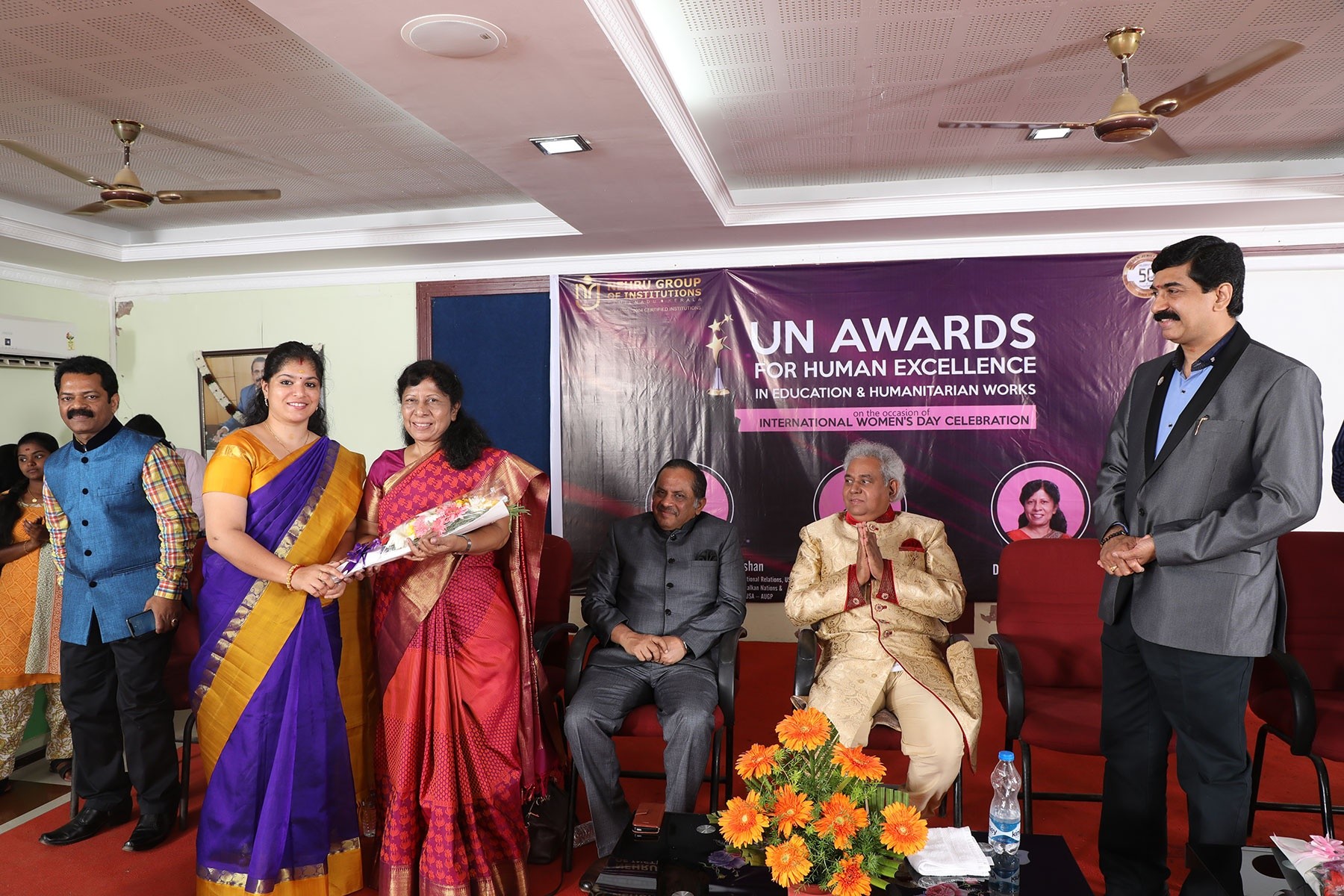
<box><xmin>379</xmin><ymin>485</ymin><xmax>531</xmax><ymax>562</ymax></box>
<box><xmin>718</xmin><ymin>706</ymin><xmax>928</xmax><ymax>896</ymax></box>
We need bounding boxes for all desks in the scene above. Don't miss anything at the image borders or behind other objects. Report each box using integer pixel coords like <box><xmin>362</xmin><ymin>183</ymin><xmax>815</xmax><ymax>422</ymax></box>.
<box><xmin>1184</xmin><ymin>842</ymin><xmax>1317</xmax><ymax>896</ymax></box>
<box><xmin>587</xmin><ymin>810</ymin><xmax>1095</xmax><ymax>896</ymax></box>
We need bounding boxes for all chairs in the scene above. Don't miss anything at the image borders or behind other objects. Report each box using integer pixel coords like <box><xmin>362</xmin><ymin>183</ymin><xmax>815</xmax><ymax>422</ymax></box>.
<box><xmin>70</xmin><ymin>539</ymin><xmax>209</xmax><ymax>834</ymax></box>
<box><xmin>530</xmin><ymin>533</ymin><xmax>748</xmax><ymax>873</ymax></box>
<box><xmin>987</xmin><ymin>538</ymin><xmax>1176</xmax><ymax>835</ymax></box>
<box><xmin>791</xmin><ymin>625</ymin><xmax>982</xmax><ymax>828</ymax></box>
<box><xmin>1247</xmin><ymin>531</ymin><xmax>1344</xmax><ymax>842</ymax></box>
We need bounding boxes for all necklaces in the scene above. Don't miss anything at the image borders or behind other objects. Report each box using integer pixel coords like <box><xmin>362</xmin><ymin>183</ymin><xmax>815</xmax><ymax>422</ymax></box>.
<box><xmin>27</xmin><ymin>487</ymin><xmax>42</xmax><ymax>503</ymax></box>
<box><xmin>266</xmin><ymin>421</ymin><xmax>309</xmax><ymax>453</ymax></box>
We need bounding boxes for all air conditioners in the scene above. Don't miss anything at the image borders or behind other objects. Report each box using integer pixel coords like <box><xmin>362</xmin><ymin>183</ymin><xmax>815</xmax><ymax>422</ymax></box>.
<box><xmin>0</xmin><ymin>313</ymin><xmax>83</xmax><ymax>369</ymax></box>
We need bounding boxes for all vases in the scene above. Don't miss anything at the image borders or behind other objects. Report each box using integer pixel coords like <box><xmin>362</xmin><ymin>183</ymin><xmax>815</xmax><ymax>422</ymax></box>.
<box><xmin>787</xmin><ymin>880</ymin><xmax>835</xmax><ymax>896</ymax></box>
<box><xmin>1311</xmin><ymin>862</ymin><xmax>1344</xmax><ymax>896</ymax></box>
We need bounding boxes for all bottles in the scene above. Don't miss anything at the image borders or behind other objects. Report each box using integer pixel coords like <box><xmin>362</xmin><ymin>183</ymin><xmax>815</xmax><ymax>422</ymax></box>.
<box><xmin>990</xmin><ymin>855</ymin><xmax>1019</xmax><ymax>896</ymax></box>
<box><xmin>988</xmin><ymin>751</ymin><xmax>1022</xmax><ymax>854</ymax></box>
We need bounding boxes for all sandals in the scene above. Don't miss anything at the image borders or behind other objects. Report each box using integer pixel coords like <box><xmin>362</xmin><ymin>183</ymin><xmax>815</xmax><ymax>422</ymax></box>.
<box><xmin>49</xmin><ymin>758</ymin><xmax>72</xmax><ymax>782</ymax></box>
<box><xmin>0</xmin><ymin>777</ymin><xmax>12</xmax><ymax>795</ymax></box>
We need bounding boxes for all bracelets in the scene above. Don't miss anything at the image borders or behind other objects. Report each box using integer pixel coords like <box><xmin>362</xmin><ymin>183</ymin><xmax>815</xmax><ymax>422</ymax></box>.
<box><xmin>24</xmin><ymin>541</ymin><xmax>28</xmax><ymax>554</ymax></box>
<box><xmin>453</xmin><ymin>534</ymin><xmax>472</xmax><ymax>555</ymax></box>
<box><xmin>683</xmin><ymin>642</ymin><xmax>689</xmax><ymax>651</ymax></box>
<box><xmin>1099</xmin><ymin>530</ymin><xmax>1129</xmax><ymax>548</ymax></box>
<box><xmin>286</xmin><ymin>564</ymin><xmax>305</xmax><ymax>593</ymax></box>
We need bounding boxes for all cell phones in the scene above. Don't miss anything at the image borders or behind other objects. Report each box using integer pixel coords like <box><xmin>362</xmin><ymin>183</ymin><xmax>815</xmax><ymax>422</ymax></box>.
<box><xmin>126</xmin><ymin>610</ymin><xmax>157</xmax><ymax>640</ymax></box>
<box><xmin>632</xmin><ymin>803</ymin><xmax>666</xmax><ymax>833</ymax></box>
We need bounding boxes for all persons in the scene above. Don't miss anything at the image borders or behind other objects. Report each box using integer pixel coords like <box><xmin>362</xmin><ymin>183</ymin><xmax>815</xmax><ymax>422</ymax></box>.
<box><xmin>563</xmin><ymin>459</ymin><xmax>747</xmax><ymax>893</ymax></box>
<box><xmin>39</xmin><ymin>355</ymin><xmax>200</xmax><ymax>852</ymax></box>
<box><xmin>212</xmin><ymin>357</ymin><xmax>265</xmax><ymax>443</ymax></box>
<box><xmin>124</xmin><ymin>414</ymin><xmax>208</xmax><ymax>539</ymax></box>
<box><xmin>0</xmin><ymin>432</ymin><xmax>74</xmax><ymax>793</ymax></box>
<box><xmin>1092</xmin><ymin>236</ymin><xmax>1323</xmax><ymax>896</ymax></box>
<box><xmin>1005</xmin><ymin>479</ymin><xmax>1072</xmax><ymax>542</ymax></box>
<box><xmin>352</xmin><ymin>359</ymin><xmax>551</xmax><ymax>896</ymax></box>
<box><xmin>784</xmin><ymin>438</ymin><xmax>982</xmax><ymax>816</ymax></box>
<box><xmin>1332</xmin><ymin>419</ymin><xmax>1344</xmax><ymax>503</ymax></box>
<box><xmin>188</xmin><ymin>341</ymin><xmax>375</xmax><ymax>896</ymax></box>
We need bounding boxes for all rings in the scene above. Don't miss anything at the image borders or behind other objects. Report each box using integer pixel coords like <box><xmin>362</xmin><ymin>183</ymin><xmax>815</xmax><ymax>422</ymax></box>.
<box><xmin>172</xmin><ymin>619</ymin><xmax>177</xmax><ymax>627</ymax></box>
<box><xmin>319</xmin><ymin>572</ymin><xmax>322</xmax><ymax>578</ymax></box>
<box><xmin>1110</xmin><ymin>566</ymin><xmax>1117</xmax><ymax>572</ymax></box>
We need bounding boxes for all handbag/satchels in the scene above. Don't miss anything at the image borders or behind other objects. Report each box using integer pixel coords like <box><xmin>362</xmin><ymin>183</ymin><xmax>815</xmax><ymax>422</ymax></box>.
<box><xmin>523</xmin><ymin>775</ymin><xmax>581</xmax><ymax>866</ymax></box>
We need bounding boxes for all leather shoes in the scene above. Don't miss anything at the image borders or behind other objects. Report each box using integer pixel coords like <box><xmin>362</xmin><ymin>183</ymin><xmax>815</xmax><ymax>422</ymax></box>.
<box><xmin>121</xmin><ymin>806</ymin><xmax>179</xmax><ymax>852</ymax></box>
<box><xmin>39</xmin><ymin>801</ymin><xmax>133</xmax><ymax>846</ymax></box>
<box><xmin>579</xmin><ymin>854</ymin><xmax>611</xmax><ymax>892</ymax></box>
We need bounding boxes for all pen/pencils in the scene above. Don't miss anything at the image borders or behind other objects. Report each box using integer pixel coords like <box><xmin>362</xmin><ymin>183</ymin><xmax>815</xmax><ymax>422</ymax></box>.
<box><xmin>1194</xmin><ymin>414</ymin><xmax>1211</xmax><ymax>436</ymax></box>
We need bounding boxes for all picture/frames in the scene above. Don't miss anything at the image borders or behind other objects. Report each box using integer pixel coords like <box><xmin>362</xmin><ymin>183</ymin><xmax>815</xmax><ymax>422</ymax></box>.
<box><xmin>196</xmin><ymin>344</ymin><xmax>326</xmax><ymax>462</ymax></box>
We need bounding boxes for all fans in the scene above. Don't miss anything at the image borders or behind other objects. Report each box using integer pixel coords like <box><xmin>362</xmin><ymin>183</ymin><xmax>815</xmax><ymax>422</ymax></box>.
<box><xmin>0</xmin><ymin>119</ymin><xmax>282</xmax><ymax>219</ymax></box>
<box><xmin>936</xmin><ymin>26</ymin><xmax>1306</xmax><ymax>163</ymax></box>
